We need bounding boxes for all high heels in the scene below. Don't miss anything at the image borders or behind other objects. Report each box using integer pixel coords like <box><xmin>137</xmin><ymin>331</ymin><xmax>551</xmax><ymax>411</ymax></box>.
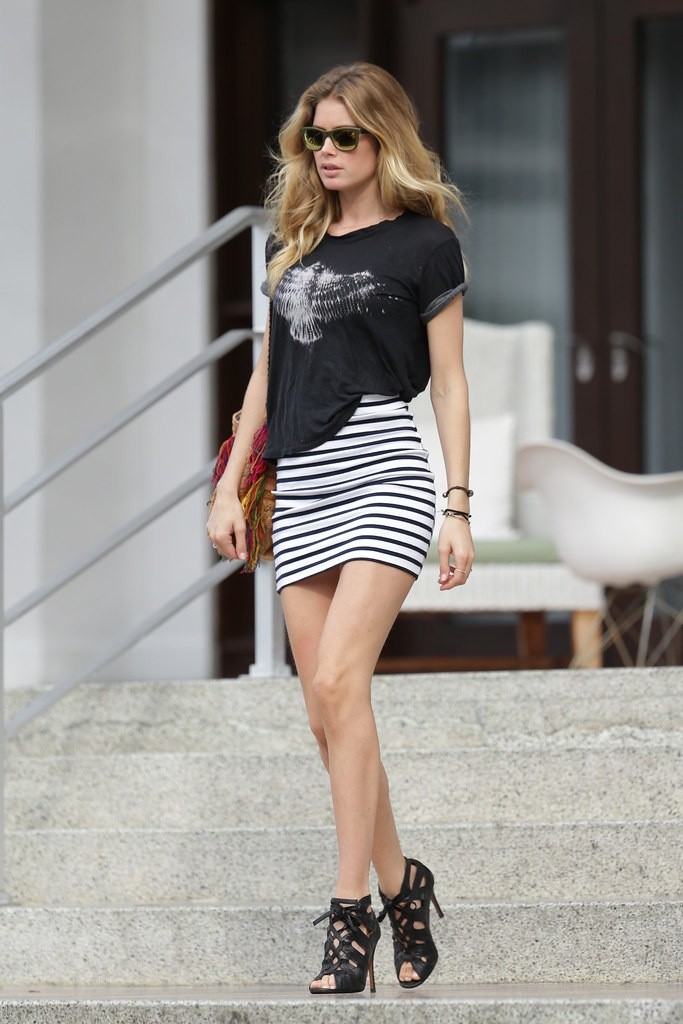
<box><xmin>377</xmin><ymin>856</ymin><xmax>444</xmax><ymax>989</ymax></box>
<box><xmin>309</xmin><ymin>894</ymin><xmax>381</xmax><ymax>994</ymax></box>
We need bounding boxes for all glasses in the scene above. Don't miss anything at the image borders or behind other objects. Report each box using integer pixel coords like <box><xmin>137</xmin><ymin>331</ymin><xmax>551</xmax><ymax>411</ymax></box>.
<box><xmin>299</xmin><ymin>125</ymin><xmax>374</xmax><ymax>152</ymax></box>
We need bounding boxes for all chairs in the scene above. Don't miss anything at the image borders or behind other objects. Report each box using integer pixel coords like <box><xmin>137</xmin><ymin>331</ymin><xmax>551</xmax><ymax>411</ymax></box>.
<box><xmin>511</xmin><ymin>437</ymin><xmax>683</xmax><ymax>667</ymax></box>
<box><xmin>374</xmin><ymin>315</ymin><xmax>609</xmax><ymax>671</ymax></box>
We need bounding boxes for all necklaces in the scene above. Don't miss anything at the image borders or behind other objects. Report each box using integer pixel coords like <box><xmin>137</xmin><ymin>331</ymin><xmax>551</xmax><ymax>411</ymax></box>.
<box><xmin>337</xmin><ymin>206</ymin><xmax>397</xmax><ymax>229</ymax></box>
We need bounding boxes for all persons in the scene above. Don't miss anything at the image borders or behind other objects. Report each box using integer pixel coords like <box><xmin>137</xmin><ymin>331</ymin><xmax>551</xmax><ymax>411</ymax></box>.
<box><xmin>202</xmin><ymin>59</ymin><xmax>477</xmax><ymax>999</ymax></box>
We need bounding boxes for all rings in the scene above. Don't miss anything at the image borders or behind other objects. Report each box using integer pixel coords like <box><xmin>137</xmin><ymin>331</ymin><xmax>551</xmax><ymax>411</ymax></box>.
<box><xmin>212</xmin><ymin>543</ymin><xmax>220</xmax><ymax>548</ymax></box>
<box><xmin>454</xmin><ymin>568</ymin><xmax>473</xmax><ymax>574</ymax></box>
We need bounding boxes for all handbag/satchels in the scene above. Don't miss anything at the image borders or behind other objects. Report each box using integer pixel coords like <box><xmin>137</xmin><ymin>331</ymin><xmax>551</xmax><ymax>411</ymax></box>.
<box><xmin>208</xmin><ymin>406</ymin><xmax>276</xmax><ymax>572</ymax></box>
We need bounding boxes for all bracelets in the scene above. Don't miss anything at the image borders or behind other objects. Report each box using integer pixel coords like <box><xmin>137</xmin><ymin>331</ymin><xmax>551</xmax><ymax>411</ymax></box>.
<box><xmin>440</xmin><ymin>508</ymin><xmax>473</xmax><ymax>526</ymax></box>
<box><xmin>441</xmin><ymin>486</ymin><xmax>474</xmax><ymax>498</ymax></box>
